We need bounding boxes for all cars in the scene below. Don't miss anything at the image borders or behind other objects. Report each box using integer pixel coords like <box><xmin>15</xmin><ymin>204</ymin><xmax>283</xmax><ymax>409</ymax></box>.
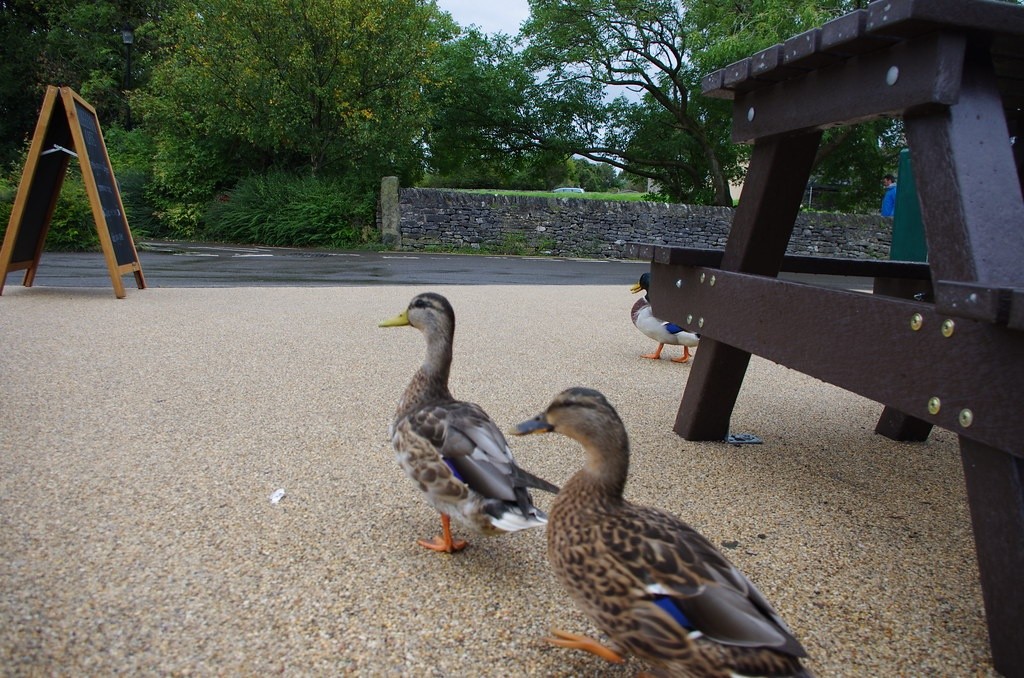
<box><xmin>551</xmin><ymin>188</ymin><xmax>585</xmax><ymax>192</ymax></box>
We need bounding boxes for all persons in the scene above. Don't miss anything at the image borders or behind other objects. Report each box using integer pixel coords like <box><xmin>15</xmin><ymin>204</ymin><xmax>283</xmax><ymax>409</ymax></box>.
<box><xmin>880</xmin><ymin>174</ymin><xmax>897</xmax><ymax>217</ymax></box>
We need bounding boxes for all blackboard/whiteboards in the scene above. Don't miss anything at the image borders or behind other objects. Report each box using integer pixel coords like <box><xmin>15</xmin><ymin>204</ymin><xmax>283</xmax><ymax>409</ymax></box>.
<box><xmin>0</xmin><ymin>85</ymin><xmax>142</xmax><ymax>276</ymax></box>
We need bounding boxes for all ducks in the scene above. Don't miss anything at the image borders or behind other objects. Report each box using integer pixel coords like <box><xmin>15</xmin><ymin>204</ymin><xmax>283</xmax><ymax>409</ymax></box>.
<box><xmin>507</xmin><ymin>386</ymin><xmax>816</xmax><ymax>678</ymax></box>
<box><xmin>376</xmin><ymin>292</ymin><xmax>559</xmax><ymax>553</ymax></box>
<box><xmin>628</xmin><ymin>273</ymin><xmax>702</xmax><ymax>362</ymax></box>
